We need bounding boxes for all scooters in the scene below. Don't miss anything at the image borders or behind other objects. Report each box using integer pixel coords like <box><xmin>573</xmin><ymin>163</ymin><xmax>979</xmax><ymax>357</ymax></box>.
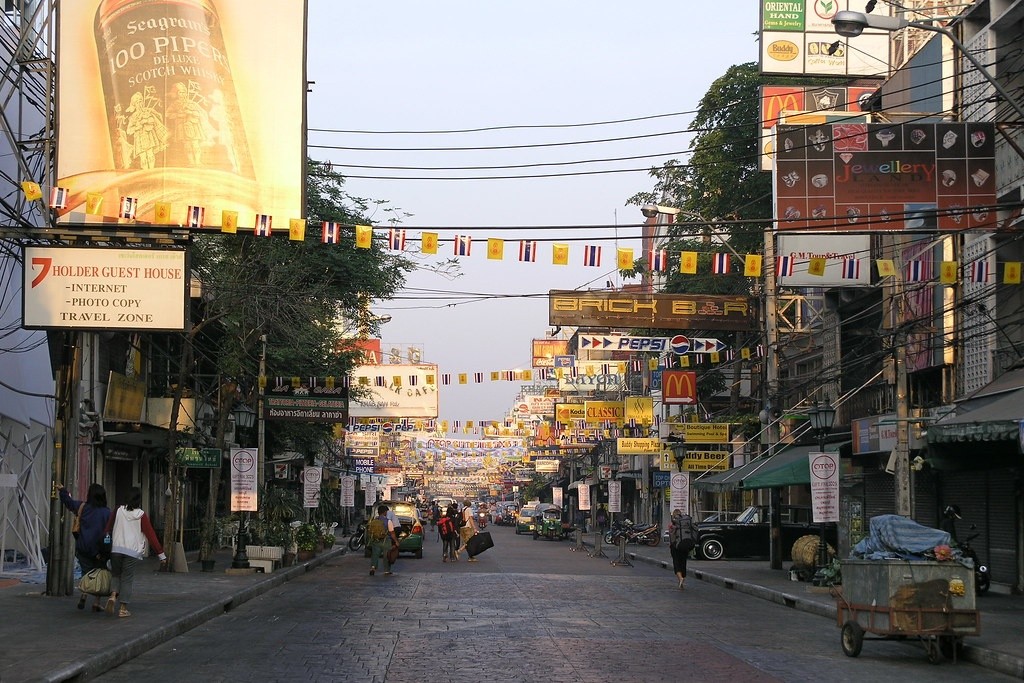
<box><xmin>956</xmin><ymin>523</ymin><xmax>991</xmax><ymax>594</ymax></box>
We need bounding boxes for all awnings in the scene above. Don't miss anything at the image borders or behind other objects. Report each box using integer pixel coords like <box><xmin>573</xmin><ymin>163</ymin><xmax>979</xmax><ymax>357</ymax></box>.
<box><xmin>568</xmin><ymin>477</ymin><xmax>600</xmax><ymax>490</ymax></box>
<box><xmin>498</xmin><ymin>493</ymin><xmax>514</xmax><ymax>500</ymax></box>
<box><xmin>694</xmin><ymin>441</ymin><xmax>852</xmax><ymax>492</ymax></box>
<box><xmin>927</xmin><ymin>365</ymin><xmax>1024</xmax><ymax>443</ymax></box>
<box><xmin>98</xmin><ymin>418</ymin><xmax>193</xmax><ymax>448</ymax></box>
<box><xmin>537</xmin><ymin>479</ymin><xmax>566</xmax><ymax>490</ymax></box>
<box><xmin>333</xmin><ymin>468</ymin><xmax>386</xmax><ymax>484</ymax></box>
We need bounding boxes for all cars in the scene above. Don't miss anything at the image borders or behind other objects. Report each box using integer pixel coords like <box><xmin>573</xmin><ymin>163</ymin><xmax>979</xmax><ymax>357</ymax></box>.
<box><xmin>364</xmin><ymin>501</ymin><xmax>428</xmax><ymax>559</ymax></box>
<box><xmin>470</xmin><ymin>499</ymin><xmax>537</xmax><ymax>534</ymax></box>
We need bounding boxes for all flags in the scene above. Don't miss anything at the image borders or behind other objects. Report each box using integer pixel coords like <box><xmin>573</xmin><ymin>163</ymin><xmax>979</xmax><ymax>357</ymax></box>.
<box><xmin>224</xmin><ymin>345</ymin><xmax>766</xmax><ymax>456</ymax></box>
<box><xmin>22</xmin><ymin>187</ymin><xmax>1022</xmax><ymax>284</ymax></box>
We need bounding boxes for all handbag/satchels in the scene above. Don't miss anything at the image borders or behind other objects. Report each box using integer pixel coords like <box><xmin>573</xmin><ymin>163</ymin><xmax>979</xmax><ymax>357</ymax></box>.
<box><xmin>72</xmin><ymin>503</ymin><xmax>85</xmax><ymax>540</ymax></box>
<box><xmin>387</xmin><ymin>545</ymin><xmax>399</xmax><ymax>564</ymax></box>
<box><xmin>78</xmin><ymin>567</ymin><xmax>111</xmax><ymax>596</ymax></box>
<box><xmin>94</xmin><ymin>534</ymin><xmax>112</xmax><ymax>559</ymax></box>
<box><xmin>465</xmin><ymin>531</ymin><xmax>495</xmax><ymax>557</ymax></box>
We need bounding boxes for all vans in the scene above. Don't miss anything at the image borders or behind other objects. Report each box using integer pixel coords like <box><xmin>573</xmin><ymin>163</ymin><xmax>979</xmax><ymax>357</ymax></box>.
<box><xmin>432</xmin><ymin>498</ymin><xmax>457</xmax><ymax>519</ymax></box>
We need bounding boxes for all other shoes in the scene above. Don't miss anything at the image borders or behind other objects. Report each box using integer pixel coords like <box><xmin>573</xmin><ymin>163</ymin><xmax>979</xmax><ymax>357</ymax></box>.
<box><xmin>443</xmin><ymin>556</ymin><xmax>448</xmax><ymax>562</ymax></box>
<box><xmin>369</xmin><ymin>568</ymin><xmax>375</xmax><ymax>576</ymax></box>
<box><xmin>678</xmin><ymin>578</ymin><xmax>684</xmax><ymax>590</ymax></box>
<box><xmin>78</xmin><ymin>594</ymin><xmax>87</xmax><ymax>609</ymax></box>
<box><xmin>455</xmin><ymin>550</ymin><xmax>462</xmax><ymax>561</ymax></box>
<box><xmin>92</xmin><ymin>604</ymin><xmax>105</xmax><ymax>612</ymax></box>
<box><xmin>468</xmin><ymin>557</ymin><xmax>478</xmax><ymax>562</ymax></box>
<box><xmin>104</xmin><ymin>598</ymin><xmax>115</xmax><ymax>617</ymax></box>
<box><xmin>385</xmin><ymin>572</ymin><xmax>392</xmax><ymax>575</ymax></box>
<box><xmin>451</xmin><ymin>558</ymin><xmax>456</xmax><ymax>562</ymax></box>
<box><xmin>118</xmin><ymin>609</ymin><xmax>131</xmax><ymax>617</ymax></box>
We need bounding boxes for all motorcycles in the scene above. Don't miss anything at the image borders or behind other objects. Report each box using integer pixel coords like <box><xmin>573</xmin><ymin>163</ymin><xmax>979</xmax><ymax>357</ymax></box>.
<box><xmin>604</xmin><ymin>518</ymin><xmax>661</xmax><ymax>546</ymax></box>
<box><xmin>532</xmin><ymin>503</ymin><xmax>564</xmax><ymax>540</ymax></box>
<box><xmin>349</xmin><ymin>520</ymin><xmax>367</xmax><ymax>551</ymax></box>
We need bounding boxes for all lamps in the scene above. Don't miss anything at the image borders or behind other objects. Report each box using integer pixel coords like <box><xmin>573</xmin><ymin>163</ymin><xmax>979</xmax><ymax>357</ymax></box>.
<box><xmin>828</xmin><ymin>0</ymin><xmax>947</xmax><ymax>71</ymax></box>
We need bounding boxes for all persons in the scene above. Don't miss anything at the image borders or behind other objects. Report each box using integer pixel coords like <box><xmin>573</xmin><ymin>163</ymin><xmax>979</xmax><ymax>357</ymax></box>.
<box><xmin>420</xmin><ymin>500</ymin><xmax>442</xmax><ymax>532</ymax></box>
<box><xmin>53</xmin><ymin>483</ymin><xmax>112</xmax><ymax>611</ymax></box>
<box><xmin>105</xmin><ymin>486</ymin><xmax>167</xmax><ymax>617</ymax></box>
<box><xmin>477</xmin><ymin>500</ymin><xmax>501</xmax><ymax>527</ymax></box>
<box><xmin>369</xmin><ymin>506</ymin><xmax>401</xmax><ymax>576</ymax></box>
<box><xmin>443</xmin><ymin>500</ymin><xmax>477</xmax><ymax>562</ymax></box>
<box><xmin>596</xmin><ymin>503</ymin><xmax>609</xmax><ymax>535</ymax></box>
<box><xmin>670</xmin><ymin>510</ymin><xmax>687</xmax><ymax>589</ymax></box>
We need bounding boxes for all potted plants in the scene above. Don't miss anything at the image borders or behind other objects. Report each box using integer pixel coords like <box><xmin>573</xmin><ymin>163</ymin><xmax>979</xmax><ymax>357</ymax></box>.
<box><xmin>282</xmin><ymin>523</ymin><xmax>335</xmax><ymax>567</ymax></box>
<box><xmin>200</xmin><ymin>517</ymin><xmax>231</xmax><ymax>572</ymax></box>
<box><xmin>245</xmin><ymin>483</ymin><xmax>307</xmax><ymax>561</ymax></box>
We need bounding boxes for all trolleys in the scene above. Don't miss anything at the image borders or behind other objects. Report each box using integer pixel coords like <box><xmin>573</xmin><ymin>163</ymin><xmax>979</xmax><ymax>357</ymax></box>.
<box><xmin>830</xmin><ymin>552</ymin><xmax>983</xmax><ymax>665</ymax></box>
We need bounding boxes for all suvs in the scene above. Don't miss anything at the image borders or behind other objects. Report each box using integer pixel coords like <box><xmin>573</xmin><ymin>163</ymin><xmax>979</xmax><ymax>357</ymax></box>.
<box><xmin>691</xmin><ymin>503</ymin><xmax>837</xmax><ymax>561</ymax></box>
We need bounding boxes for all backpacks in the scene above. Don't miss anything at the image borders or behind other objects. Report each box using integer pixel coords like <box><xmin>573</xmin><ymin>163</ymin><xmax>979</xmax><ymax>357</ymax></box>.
<box><xmin>436</xmin><ymin>519</ymin><xmax>452</xmax><ymax>540</ymax></box>
<box><xmin>455</xmin><ymin>506</ymin><xmax>470</xmax><ymax>527</ymax></box>
<box><xmin>672</xmin><ymin>514</ymin><xmax>693</xmax><ymax>553</ymax></box>
<box><xmin>370</xmin><ymin>516</ymin><xmax>388</xmax><ymax>539</ymax></box>
